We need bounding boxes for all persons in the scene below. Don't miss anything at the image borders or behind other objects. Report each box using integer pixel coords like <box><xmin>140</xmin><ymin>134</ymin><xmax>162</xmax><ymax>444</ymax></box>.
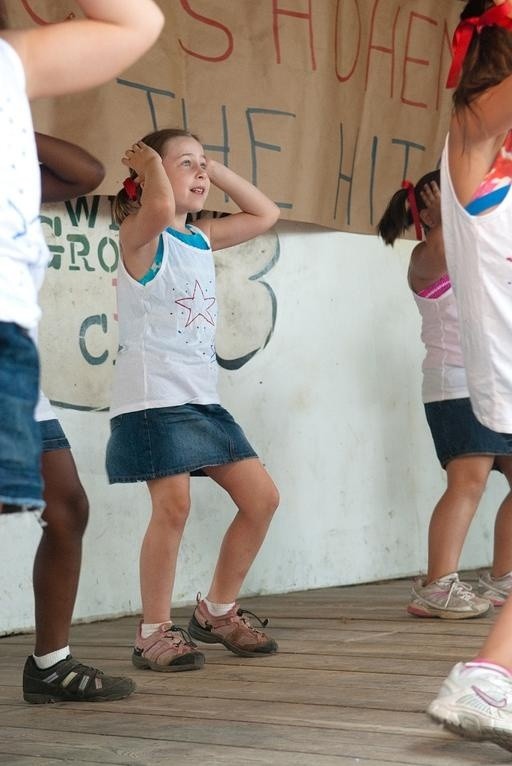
<box><xmin>17</xmin><ymin>130</ymin><xmax>136</xmax><ymax>703</ymax></box>
<box><xmin>0</xmin><ymin>2</ymin><xmax>171</xmax><ymax>503</ymax></box>
<box><xmin>109</xmin><ymin>129</ymin><xmax>285</xmax><ymax>676</ymax></box>
<box><xmin>380</xmin><ymin>170</ymin><xmax>511</xmax><ymax>618</ymax></box>
<box><xmin>420</xmin><ymin>0</ymin><xmax>512</xmax><ymax>766</ymax></box>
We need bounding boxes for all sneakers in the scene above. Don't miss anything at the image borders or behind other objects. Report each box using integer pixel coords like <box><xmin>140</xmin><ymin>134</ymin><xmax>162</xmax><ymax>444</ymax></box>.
<box><xmin>426</xmin><ymin>660</ymin><xmax>512</xmax><ymax>751</ymax></box>
<box><xmin>23</xmin><ymin>655</ymin><xmax>136</xmax><ymax>704</ymax></box>
<box><xmin>408</xmin><ymin>567</ymin><xmax>512</xmax><ymax>620</ymax></box>
<box><xmin>132</xmin><ymin>591</ymin><xmax>277</xmax><ymax>673</ymax></box>
